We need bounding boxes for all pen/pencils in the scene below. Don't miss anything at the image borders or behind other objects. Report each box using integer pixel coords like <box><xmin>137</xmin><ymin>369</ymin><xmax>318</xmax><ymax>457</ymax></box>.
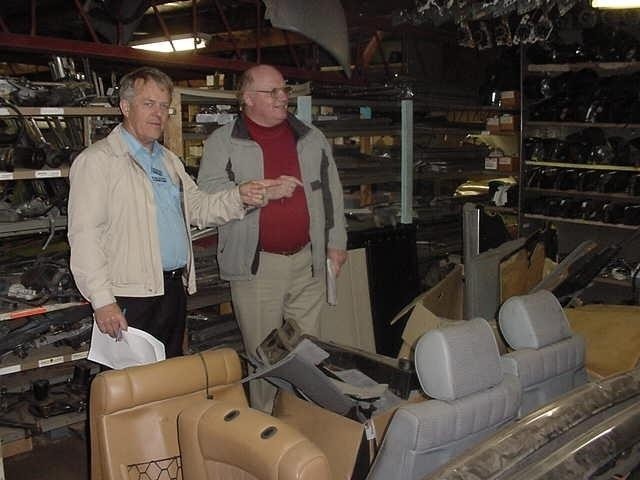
<box><xmin>115</xmin><ymin>307</ymin><xmax>127</xmax><ymax>341</ymax></box>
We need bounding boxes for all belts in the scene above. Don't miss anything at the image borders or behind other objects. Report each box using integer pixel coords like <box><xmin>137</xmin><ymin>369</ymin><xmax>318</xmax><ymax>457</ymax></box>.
<box><xmin>163</xmin><ymin>270</ymin><xmax>186</xmax><ymax>280</ymax></box>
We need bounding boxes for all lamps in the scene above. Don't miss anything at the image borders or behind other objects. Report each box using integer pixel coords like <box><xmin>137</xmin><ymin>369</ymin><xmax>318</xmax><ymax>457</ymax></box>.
<box><xmin>130</xmin><ymin>32</ymin><xmax>213</xmax><ymax>54</ymax></box>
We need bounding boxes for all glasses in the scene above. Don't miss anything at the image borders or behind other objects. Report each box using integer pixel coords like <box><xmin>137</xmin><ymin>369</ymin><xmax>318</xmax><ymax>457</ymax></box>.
<box><xmin>246</xmin><ymin>86</ymin><xmax>292</xmax><ymax>99</ymax></box>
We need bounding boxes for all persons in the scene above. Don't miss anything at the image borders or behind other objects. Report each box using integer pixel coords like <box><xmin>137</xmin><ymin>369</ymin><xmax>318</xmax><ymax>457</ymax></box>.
<box><xmin>197</xmin><ymin>64</ymin><xmax>350</xmax><ymax>414</ymax></box>
<box><xmin>64</xmin><ymin>66</ymin><xmax>267</xmax><ymax>375</ymax></box>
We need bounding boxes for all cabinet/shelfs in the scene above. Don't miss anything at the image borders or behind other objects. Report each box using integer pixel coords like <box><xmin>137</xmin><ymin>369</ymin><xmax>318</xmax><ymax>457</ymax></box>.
<box><xmin>404</xmin><ymin>93</ymin><xmax>518</xmax><ymax>300</ymax></box>
<box><xmin>305</xmin><ymin>91</ymin><xmax>403</xmax><ymax>315</ymax></box>
<box><xmin>521</xmin><ymin>58</ymin><xmax>640</xmax><ymax>308</ymax></box>
<box><xmin>1</xmin><ymin>105</ymin><xmax>158</xmax><ymax>458</ymax></box>
<box><xmin>162</xmin><ymin>86</ymin><xmax>303</xmax><ymax>354</ymax></box>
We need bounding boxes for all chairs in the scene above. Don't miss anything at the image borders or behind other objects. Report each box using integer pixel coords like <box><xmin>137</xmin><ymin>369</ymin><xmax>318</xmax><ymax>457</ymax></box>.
<box><xmin>495</xmin><ymin>288</ymin><xmax>585</xmax><ymax>405</ymax></box>
<box><xmin>368</xmin><ymin>318</ymin><xmax>519</xmax><ymax>480</ymax></box>
